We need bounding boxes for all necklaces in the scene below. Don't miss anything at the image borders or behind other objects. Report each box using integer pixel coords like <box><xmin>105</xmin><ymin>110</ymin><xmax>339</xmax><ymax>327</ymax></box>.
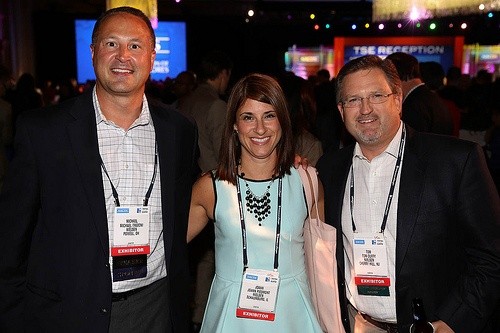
<box><xmin>239</xmin><ymin>166</ymin><xmax>277</xmax><ymax>227</ymax></box>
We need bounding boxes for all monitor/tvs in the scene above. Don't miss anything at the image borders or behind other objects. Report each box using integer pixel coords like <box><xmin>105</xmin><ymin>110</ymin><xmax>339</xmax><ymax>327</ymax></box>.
<box><xmin>74</xmin><ymin>19</ymin><xmax>187</xmax><ymax>85</ymax></box>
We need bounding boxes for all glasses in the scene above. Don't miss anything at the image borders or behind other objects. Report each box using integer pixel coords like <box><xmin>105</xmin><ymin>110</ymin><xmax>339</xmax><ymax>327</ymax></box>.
<box><xmin>340</xmin><ymin>91</ymin><xmax>398</xmax><ymax>107</ymax></box>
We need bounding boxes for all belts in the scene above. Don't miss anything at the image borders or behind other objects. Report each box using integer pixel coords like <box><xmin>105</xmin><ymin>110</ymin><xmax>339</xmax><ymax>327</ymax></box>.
<box><xmin>350</xmin><ymin>302</ymin><xmax>397</xmax><ymax>333</ymax></box>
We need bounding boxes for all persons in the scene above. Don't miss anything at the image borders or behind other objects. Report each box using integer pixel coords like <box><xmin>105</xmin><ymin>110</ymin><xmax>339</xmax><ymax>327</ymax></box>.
<box><xmin>187</xmin><ymin>72</ymin><xmax>324</xmax><ymax>333</ymax></box>
<box><xmin>167</xmin><ymin>48</ymin><xmax>499</xmax><ymax>173</ymax></box>
<box><xmin>0</xmin><ymin>7</ymin><xmax>199</xmax><ymax>333</ymax></box>
<box><xmin>315</xmin><ymin>54</ymin><xmax>500</xmax><ymax>333</ymax></box>
<box><xmin>0</xmin><ymin>63</ymin><xmax>200</xmax><ymax>187</ymax></box>
